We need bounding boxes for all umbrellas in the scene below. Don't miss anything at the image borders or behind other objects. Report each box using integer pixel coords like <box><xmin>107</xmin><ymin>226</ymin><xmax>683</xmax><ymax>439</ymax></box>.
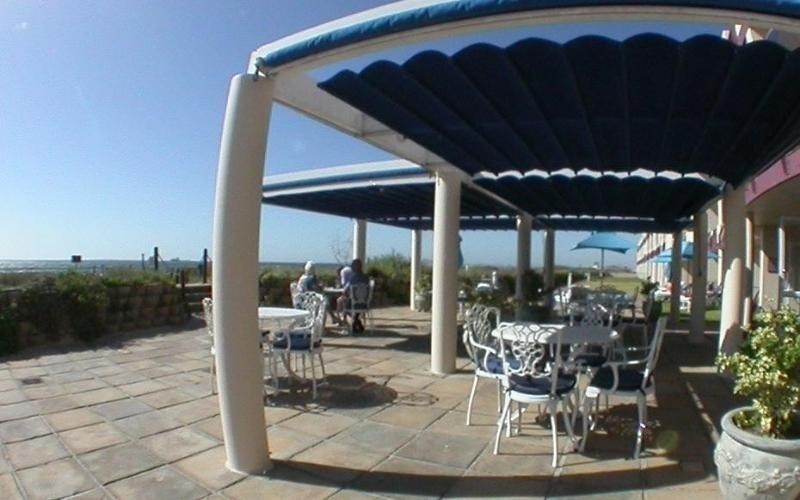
<box><xmin>650</xmin><ymin>241</ymin><xmax>720</xmax><ymax>283</ymax></box>
<box><xmin>570</xmin><ymin>232</ymin><xmax>633</xmax><ymax>276</ymax></box>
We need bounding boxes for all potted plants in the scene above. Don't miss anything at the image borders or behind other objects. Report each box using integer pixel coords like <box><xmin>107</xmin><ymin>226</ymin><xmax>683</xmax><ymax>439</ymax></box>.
<box><xmin>413</xmin><ymin>274</ymin><xmax>432</xmax><ymax>312</ymax></box>
<box><xmin>714</xmin><ymin>304</ymin><xmax>800</xmax><ymax>500</ymax></box>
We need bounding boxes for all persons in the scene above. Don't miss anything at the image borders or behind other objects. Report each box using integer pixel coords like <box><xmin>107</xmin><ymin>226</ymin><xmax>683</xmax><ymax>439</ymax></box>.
<box><xmin>297</xmin><ymin>259</ymin><xmax>368</xmax><ymax>331</ymax></box>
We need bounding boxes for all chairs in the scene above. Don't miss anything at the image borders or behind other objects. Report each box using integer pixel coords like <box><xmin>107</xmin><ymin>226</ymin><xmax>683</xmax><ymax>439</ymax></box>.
<box><xmin>462</xmin><ymin>280</ymin><xmax>670</xmax><ymax>462</ymax></box>
<box><xmin>201</xmin><ymin>277</ymin><xmax>376</xmax><ymax>399</ymax></box>
